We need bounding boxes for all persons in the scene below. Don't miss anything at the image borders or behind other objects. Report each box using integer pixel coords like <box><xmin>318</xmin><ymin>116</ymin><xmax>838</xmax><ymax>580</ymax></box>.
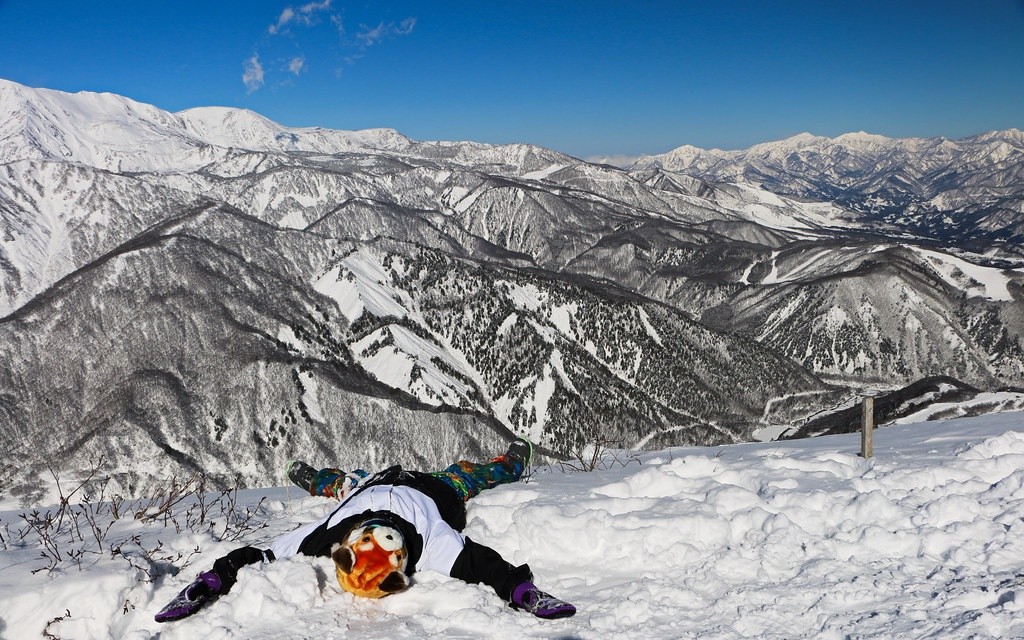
<box><xmin>155</xmin><ymin>437</ymin><xmax>576</xmax><ymax>623</ymax></box>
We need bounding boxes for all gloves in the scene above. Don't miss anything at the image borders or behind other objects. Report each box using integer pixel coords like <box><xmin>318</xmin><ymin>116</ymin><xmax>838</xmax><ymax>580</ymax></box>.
<box><xmin>508</xmin><ymin>578</ymin><xmax>576</xmax><ymax>620</ymax></box>
<box><xmin>153</xmin><ymin>570</ymin><xmax>225</xmax><ymax>623</ymax></box>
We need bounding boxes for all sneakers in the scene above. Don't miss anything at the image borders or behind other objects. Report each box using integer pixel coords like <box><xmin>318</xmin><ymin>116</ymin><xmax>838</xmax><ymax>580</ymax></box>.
<box><xmin>505</xmin><ymin>435</ymin><xmax>533</xmax><ymax>481</ymax></box>
<box><xmin>283</xmin><ymin>459</ymin><xmax>319</xmax><ymax>493</ymax></box>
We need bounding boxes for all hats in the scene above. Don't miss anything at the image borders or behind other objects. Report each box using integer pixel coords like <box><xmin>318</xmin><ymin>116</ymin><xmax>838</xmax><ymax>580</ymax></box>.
<box><xmin>331</xmin><ymin>518</ymin><xmax>410</xmax><ymax>600</ymax></box>
